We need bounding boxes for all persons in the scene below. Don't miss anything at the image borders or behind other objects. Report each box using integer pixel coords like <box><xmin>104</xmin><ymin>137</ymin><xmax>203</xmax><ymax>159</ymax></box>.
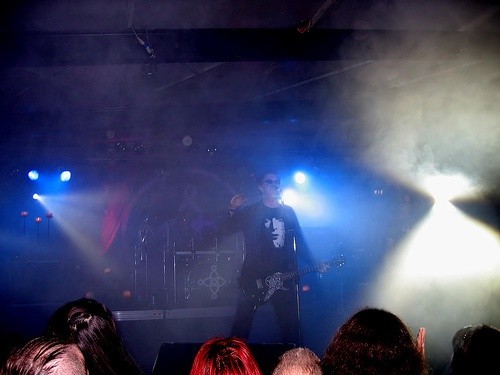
<box><xmin>191</xmin><ymin>309</ymin><xmax>500</xmax><ymax>375</ymax></box>
<box><xmin>223</xmin><ymin>170</ymin><xmax>328</xmax><ymax>347</ymax></box>
<box><xmin>1</xmin><ymin>297</ymin><xmax>143</xmax><ymax>375</ymax></box>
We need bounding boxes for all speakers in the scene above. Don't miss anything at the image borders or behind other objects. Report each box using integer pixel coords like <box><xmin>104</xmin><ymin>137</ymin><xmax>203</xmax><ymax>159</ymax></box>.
<box><xmin>150</xmin><ymin>342</ymin><xmax>296</xmax><ymax>375</ymax></box>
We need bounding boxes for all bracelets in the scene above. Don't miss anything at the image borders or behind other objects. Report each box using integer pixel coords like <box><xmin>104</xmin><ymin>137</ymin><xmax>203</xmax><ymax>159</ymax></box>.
<box><xmin>229</xmin><ymin>208</ymin><xmax>237</xmax><ymax>212</ymax></box>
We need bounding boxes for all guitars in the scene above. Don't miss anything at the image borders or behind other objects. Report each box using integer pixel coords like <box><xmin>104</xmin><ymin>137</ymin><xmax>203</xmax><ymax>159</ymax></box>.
<box><xmin>235</xmin><ymin>253</ymin><xmax>343</xmax><ymax>309</ymax></box>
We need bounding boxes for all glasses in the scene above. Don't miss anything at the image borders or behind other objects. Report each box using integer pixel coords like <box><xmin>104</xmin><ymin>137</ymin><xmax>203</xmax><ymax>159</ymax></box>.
<box><xmin>266</xmin><ymin>180</ymin><xmax>281</xmax><ymax>184</ymax></box>
